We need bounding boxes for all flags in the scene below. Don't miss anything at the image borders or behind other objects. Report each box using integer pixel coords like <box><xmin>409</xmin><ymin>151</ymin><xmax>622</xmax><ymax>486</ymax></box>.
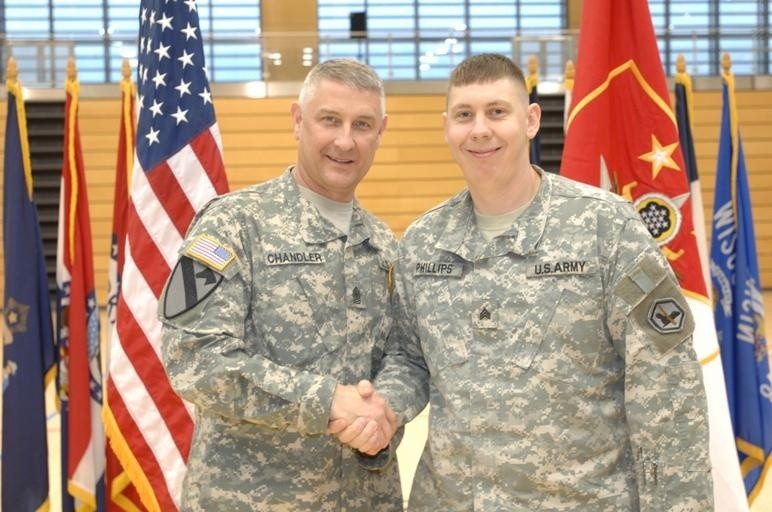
<box><xmin>556</xmin><ymin>1</ymin><xmax>749</xmax><ymax>512</ymax></box>
<box><xmin>563</xmin><ymin>59</ymin><xmax>576</xmax><ymax>135</ymax></box>
<box><xmin>671</xmin><ymin>54</ymin><xmax>711</xmax><ymax>292</ymax></box>
<box><xmin>103</xmin><ymin>0</ymin><xmax>230</xmax><ymax>512</ymax></box>
<box><xmin>52</xmin><ymin>61</ymin><xmax>106</xmax><ymax>512</ymax></box>
<box><xmin>708</xmin><ymin>55</ymin><xmax>772</xmax><ymax>505</ymax></box>
<box><xmin>525</xmin><ymin>54</ymin><xmax>543</xmax><ymax>167</ymax></box>
<box><xmin>0</xmin><ymin>57</ymin><xmax>59</xmax><ymax>512</ymax></box>
<box><xmin>105</xmin><ymin>58</ymin><xmax>139</xmax><ymax>347</ymax></box>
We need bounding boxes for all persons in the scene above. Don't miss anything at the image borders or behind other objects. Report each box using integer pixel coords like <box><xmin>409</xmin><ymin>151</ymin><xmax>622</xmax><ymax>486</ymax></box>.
<box><xmin>327</xmin><ymin>53</ymin><xmax>713</xmax><ymax>511</ymax></box>
<box><xmin>154</xmin><ymin>56</ymin><xmax>408</xmax><ymax>511</ymax></box>
